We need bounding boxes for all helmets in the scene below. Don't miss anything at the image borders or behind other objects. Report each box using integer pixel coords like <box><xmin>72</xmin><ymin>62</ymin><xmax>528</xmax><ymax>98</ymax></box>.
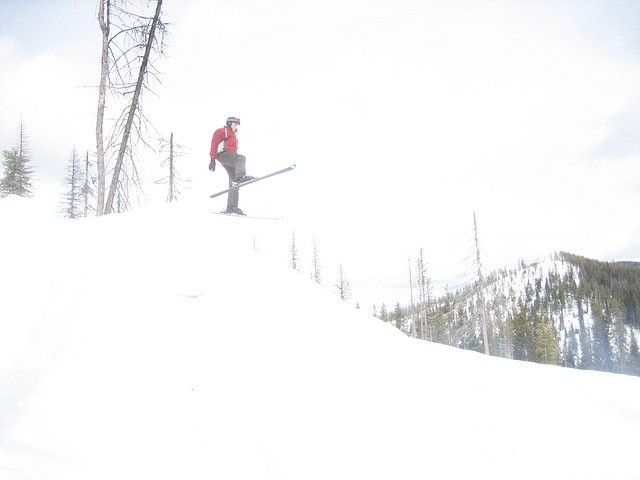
<box><xmin>226</xmin><ymin>117</ymin><xmax>240</xmax><ymax>126</ymax></box>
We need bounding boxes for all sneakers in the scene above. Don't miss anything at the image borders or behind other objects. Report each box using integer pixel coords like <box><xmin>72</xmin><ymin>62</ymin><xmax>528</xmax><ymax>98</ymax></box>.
<box><xmin>226</xmin><ymin>207</ymin><xmax>243</xmax><ymax>215</ymax></box>
<box><xmin>237</xmin><ymin>175</ymin><xmax>255</xmax><ymax>183</ymax></box>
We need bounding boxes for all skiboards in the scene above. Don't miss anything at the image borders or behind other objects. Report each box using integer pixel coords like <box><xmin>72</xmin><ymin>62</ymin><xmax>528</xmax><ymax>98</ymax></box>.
<box><xmin>210</xmin><ymin>164</ymin><xmax>297</xmax><ymax>220</ymax></box>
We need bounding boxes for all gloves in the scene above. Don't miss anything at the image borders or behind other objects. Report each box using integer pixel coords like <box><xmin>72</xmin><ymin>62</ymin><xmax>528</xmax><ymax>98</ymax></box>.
<box><xmin>209</xmin><ymin>160</ymin><xmax>215</xmax><ymax>171</ymax></box>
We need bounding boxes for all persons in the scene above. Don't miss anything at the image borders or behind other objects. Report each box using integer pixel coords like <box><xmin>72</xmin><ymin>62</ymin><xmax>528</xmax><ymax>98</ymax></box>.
<box><xmin>209</xmin><ymin>116</ymin><xmax>259</xmax><ymax>219</ymax></box>
